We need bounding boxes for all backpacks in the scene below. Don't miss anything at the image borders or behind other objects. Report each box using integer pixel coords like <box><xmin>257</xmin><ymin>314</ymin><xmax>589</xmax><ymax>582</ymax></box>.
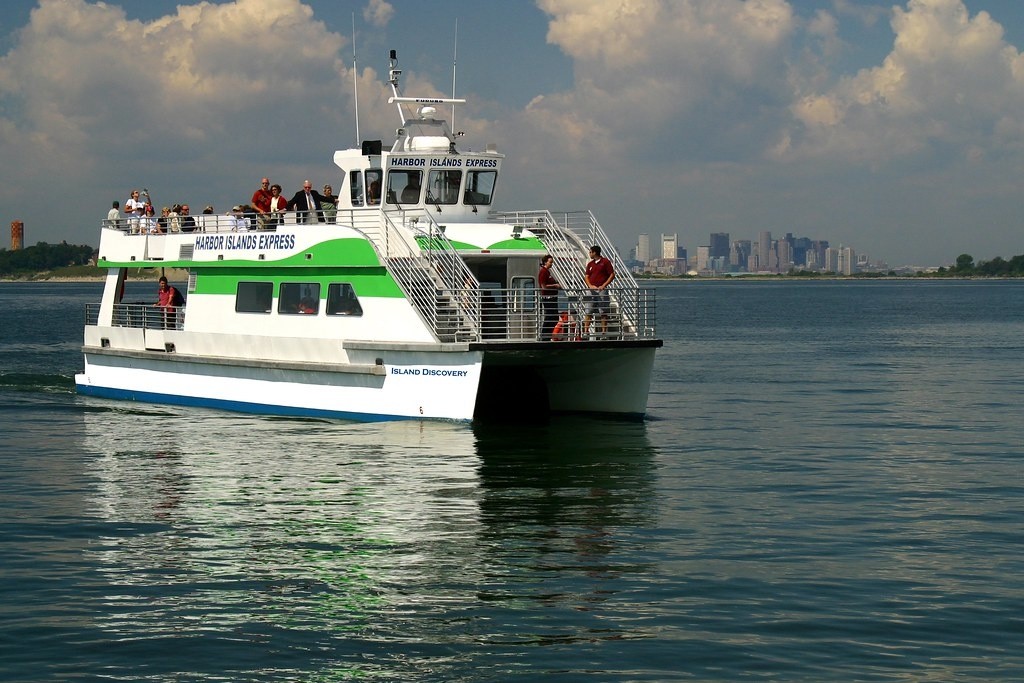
<box><xmin>168</xmin><ymin>285</ymin><xmax>184</xmax><ymax>306</ymax></box>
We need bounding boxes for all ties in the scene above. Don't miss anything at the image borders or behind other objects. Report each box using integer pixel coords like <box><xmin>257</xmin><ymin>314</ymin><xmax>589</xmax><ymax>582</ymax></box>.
<box><xmin>308</xmin><ymin>195</ymin><xmax>312</xmax><ymax>209</ymax></box>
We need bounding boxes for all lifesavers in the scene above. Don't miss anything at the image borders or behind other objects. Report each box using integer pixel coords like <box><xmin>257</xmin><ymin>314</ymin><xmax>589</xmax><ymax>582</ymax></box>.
<box><xmin>552</xmin><ymin>319</ymin><xmax>582</xmax><ymax>341</ymax></box>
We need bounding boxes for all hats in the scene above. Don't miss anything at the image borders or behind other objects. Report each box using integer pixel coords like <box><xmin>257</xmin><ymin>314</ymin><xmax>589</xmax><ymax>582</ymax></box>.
<box><xmin>232</xmin><ymin>205</ymin><xmax>240</xmax><ymax>211</ymax></box>
<box><xmin>137</xmin><ymin>205</ymin><xmax>145</xmax><ymax>210</ymax></box>
<box><xmin>146</xmin><ymin>205</ymin><xmax>154</xmax><ymax>211</ymax></box>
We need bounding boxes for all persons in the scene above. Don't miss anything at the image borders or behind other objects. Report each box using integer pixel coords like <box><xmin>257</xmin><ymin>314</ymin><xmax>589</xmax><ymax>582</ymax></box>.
<box><xmin>152</xmin><ymin>276</ymin><xmax>176</xmax><ymax>329</ymax></box>
<box><xmin>332</xmin><ymin>290</ymin><xmax>361</xmax><ymax>314</ymax></box>
<box><xmin>203</xmin><ymin>205</ymin><xmax>214</xmax><ymax>214</ymax></box>
<box><xmin>459</xmin><ymin>276</ymin><xmax>496</xmax><ymax>329</ymax></box>
<box><xmin>582</xmin><ymin>246</ymin><xmax>615</xmax><ymax>340</ymax></box>
<box><xmin>401</xmin><ymin>172</ymin><xmax>420</xmax><ymax>203</ymax></box>
<box><xmin>537</xmin><ymin>254</ymin><xmax>561</xmax><ymax>341</ymax></box>
<box><xmin>106</xmin><ymin>191</ymin><xmax>202</xmax><ymax>234</ymax></box>
<box><xmin>297</xmin><ymin>287</ymin><xmax>318</xmax><ymax>314</ymax></box>
<box><xmin>229</xmin><ymin>178</ymin><xmax>337</xmax><ymax>233</ymax></box>
<box><xmin>367</xmin><ymin>181</ymin><xmax>380</xmax><ymax>205</ymax></box>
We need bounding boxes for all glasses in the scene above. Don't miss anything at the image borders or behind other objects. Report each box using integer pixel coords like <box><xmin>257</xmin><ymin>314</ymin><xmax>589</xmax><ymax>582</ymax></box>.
<box><xmin>182</xmin><ymin>209</ymin><xmax>189</xmax><ymax>211</ymax></box>
<box><xmin>305</xmin><ymin>186</ymin><xmax>311</xmax><ymax>189</ymax></box>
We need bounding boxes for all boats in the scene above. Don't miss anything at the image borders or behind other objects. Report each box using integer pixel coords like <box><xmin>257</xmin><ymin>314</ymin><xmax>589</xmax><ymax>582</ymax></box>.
<box><xmin>75</xmin><ymin>10</ymin><xmax>666</xmax><ymax>424</ymax></box>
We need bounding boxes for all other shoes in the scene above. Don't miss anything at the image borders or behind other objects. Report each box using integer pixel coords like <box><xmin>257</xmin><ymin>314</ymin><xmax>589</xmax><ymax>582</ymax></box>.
<box><xmin>600</xmin><ymin>336</ymin><xmax>609</xmax><ymax>340</ymax></box>
<box><xmin>582</xmin><ymin>333</ymin><xmax>589</xmax><ymax>341</ymax></box>
